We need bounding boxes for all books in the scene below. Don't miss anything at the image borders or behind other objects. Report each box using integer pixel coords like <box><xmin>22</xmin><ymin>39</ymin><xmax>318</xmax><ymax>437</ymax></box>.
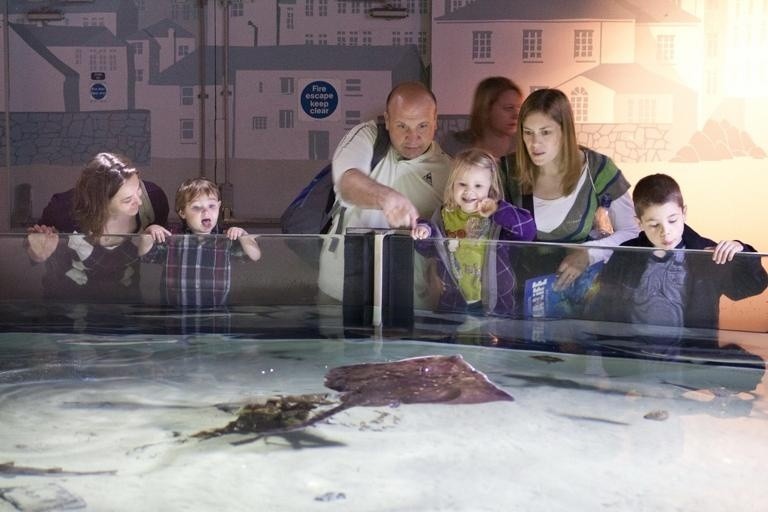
<box><xmin>523</xmin><ymin>258</ymin><xmax>605</xmax><ymax>344</ymax></box>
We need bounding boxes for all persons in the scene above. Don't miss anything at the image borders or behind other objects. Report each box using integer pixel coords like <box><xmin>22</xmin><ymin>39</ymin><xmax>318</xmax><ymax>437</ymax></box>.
<box><xmin>48</xmin><ymin>304</ymin><xmax>144</xmax><ymax>381</ymax></box>
<box><xmin>151</xmin><ymin>306</ymin><xmax>257</xmax><ymax>395</ymax></box>
<box><xmin>587</xmin><ymin>172</ymin><xmax>766</xmax><ymax>346</ymax></box>
<box><xmin>487</xmin><ymin>87</ymin><xmax>644</xmax><ymax>294</ymax></box>
<box><xmin>312</xmin><ymin>82</ymin><xmax>464</xmax><ymax>335</ymax></box>
<box><xmin>408</xmin><ymin>148</ymin><xmax>536</xmax><ymax>315</ymax></box>
<box><xmin>20</xmin><ymin>152</ymin><xmax>169</xmax><ymax>303</ymax></box>
<box><xmin>141</xmin><ymin>177</ymin><xmax>261</xmax><ymax>305</ymax></box>
<box><xmin>444</xmin><ymin>75</ymin><xmax>528</xmax><ymax>155</ymax></box>
<box><xmin>602</xmin><ymin>347</ymin><xmax>767</xmax><ymax>421</ymax></box>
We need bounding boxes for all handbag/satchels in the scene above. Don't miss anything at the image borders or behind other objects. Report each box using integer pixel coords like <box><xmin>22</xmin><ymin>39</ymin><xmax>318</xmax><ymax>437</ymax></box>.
<box><xmin>282</xmin><ymin>164</ymin><xmax>335</xmax><ymax>269</ymax></box>
